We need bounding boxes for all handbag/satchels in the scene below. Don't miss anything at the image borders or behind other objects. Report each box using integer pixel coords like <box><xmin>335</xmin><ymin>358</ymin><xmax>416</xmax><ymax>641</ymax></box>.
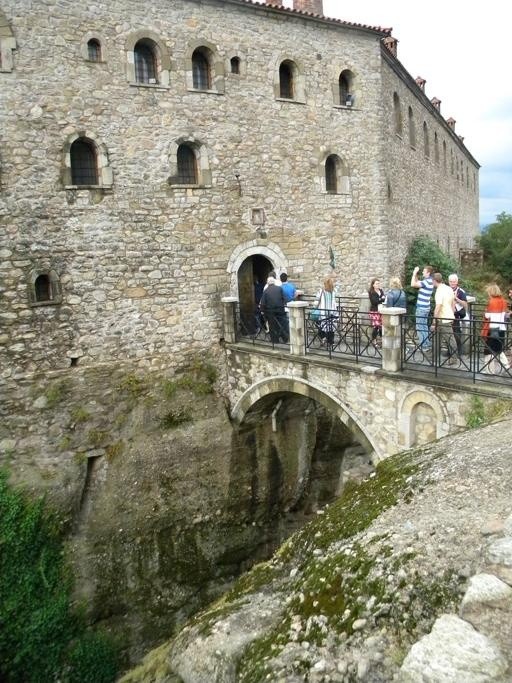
<box><xmin>454</xmin><ymin>307</ymin><xmax>467</xmax><ymax>320</ymax></box>
<box><xmin>487</xmin><ymin>327</ymin><xmax>500</xmax><ymax>343</ymax></box>
<box><xmin>310</xmin><ymin>308</ymin><xmax>321</xmax><ymax>321</ymax></box>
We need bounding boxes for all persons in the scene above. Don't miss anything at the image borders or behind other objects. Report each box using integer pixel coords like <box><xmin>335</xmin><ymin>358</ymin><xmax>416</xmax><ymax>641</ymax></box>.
<box><xmin>479</xmin><ymin>280</ymin><xmax>511</xmax><ymax>377</ymax></box>
<box><xmin>255</xmin><ymin>261</ymin><xmax>476</xmax><ymax>367</ymax></box>
